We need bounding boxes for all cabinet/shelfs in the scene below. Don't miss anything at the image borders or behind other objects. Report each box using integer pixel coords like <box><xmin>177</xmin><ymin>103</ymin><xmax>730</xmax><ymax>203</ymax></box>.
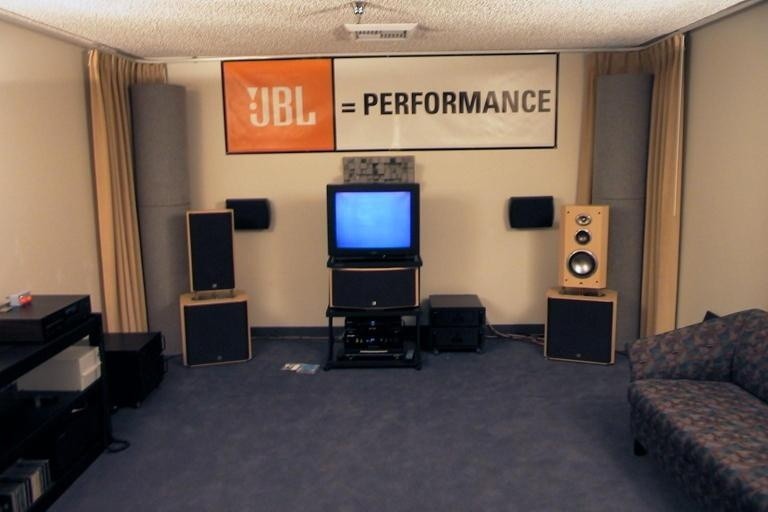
<box><xmin>426</xmin><ymin>293</ymin><xmax>487</xmax><ymax>356</ymax></box>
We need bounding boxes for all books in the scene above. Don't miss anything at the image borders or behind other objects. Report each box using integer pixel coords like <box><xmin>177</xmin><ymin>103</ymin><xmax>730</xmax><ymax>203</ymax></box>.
<box><xmin>0</xmin><ymin>459</ymin><xmax>52</xmax><ymax>512</ymax></box>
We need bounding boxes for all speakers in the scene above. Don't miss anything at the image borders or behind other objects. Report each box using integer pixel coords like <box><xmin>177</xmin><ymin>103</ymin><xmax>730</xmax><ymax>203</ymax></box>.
<box><xmin>544</xmin><ymin>288</ymin><xmax>618</xmax><ymax>364</ymax></box>
<box><xmin>507</xmin><ymin>195</ymin><xmax>555</xmax><ymax>229</ymax></box>
<box><xmin>185</xmin><ymin>209</ymin><xmax>235</xmax><ymax>295</ymax></box>
<box><xmin>332</xmin><ymin>268</ymin><xmax>422</xmax><ymax>307</ymax></box>
<box><xmin>178</xmin><ymin>292</ymin><xmax>253</xmax><ymax>368</ymax></box>
<box><xmin>226</xmin><ymin>198</ymin><xmax>271</xmax><ymax>231</ymax></box>
<box><xmin>556</xmin><ymin>203</ymin><xmax>611</xmax><ymax>298</ymax></box>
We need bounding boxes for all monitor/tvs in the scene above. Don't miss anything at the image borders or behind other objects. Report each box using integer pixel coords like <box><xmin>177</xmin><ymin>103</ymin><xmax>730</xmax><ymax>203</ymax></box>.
<box><xmin>325</xmin><ymin>182</ymin><xmax>422</xmax><ymax>267</ymax></box>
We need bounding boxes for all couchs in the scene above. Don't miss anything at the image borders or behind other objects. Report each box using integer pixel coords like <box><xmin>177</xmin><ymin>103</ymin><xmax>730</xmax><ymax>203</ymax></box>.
<box><xmin>625</xmin><ymin>303</ymin><xmax>768</xmax><ymax>512</ymax></box>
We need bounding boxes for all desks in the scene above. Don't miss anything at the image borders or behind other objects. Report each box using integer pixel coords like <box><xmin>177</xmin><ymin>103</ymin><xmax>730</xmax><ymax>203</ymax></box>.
<box><xmin>1</xmin><ymin>313</ymin><xmax>115</xmax><ymax>511</ymax></box>
